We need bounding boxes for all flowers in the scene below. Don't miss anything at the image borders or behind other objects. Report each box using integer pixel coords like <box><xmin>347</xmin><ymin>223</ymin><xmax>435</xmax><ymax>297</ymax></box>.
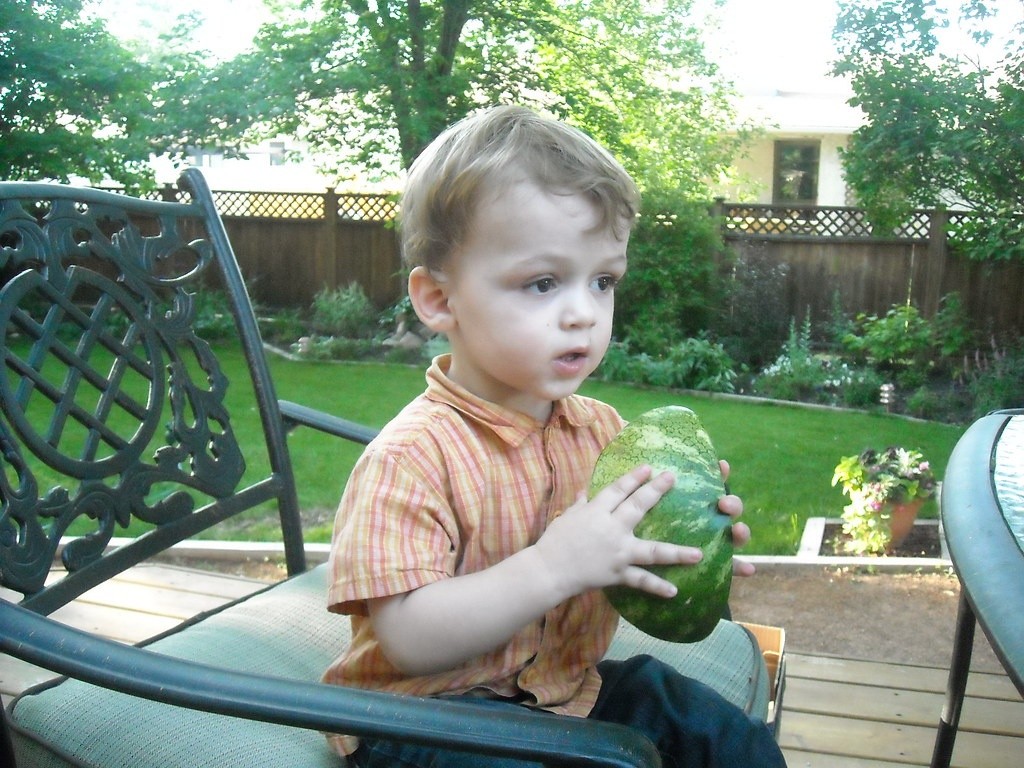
<box><xmin>831</xmin><ymin>446</ymin><xmax>941</xmax><ymax>555</ymax></box>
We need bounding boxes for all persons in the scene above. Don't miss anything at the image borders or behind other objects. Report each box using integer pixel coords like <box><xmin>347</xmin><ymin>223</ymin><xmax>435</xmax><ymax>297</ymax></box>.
<box><xmin>319</xmin><ymin>106</ymin><xmax>783</xmax><ymax>768</ymax></box>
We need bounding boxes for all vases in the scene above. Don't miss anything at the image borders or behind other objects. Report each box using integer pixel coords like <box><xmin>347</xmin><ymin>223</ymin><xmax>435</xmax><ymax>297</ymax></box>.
<box><xmin>888</xmin><ymin>498</ymin><xmax>924</xmax><ymax>549</ymax></box>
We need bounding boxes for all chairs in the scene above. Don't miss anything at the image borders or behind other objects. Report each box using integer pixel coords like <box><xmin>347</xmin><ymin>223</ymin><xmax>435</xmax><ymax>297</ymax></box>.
<box><xmin>0</xmin><ymin>167</ymin><xmax>785</xmax><ymax>768</ymax></box>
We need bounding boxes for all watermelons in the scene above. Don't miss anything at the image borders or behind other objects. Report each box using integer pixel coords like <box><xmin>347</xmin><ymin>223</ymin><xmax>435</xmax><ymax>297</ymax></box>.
<box><xmin>585</xmin><ymin>406</ymin><xmax>733</xmax><ymax>643</ymax></box>
